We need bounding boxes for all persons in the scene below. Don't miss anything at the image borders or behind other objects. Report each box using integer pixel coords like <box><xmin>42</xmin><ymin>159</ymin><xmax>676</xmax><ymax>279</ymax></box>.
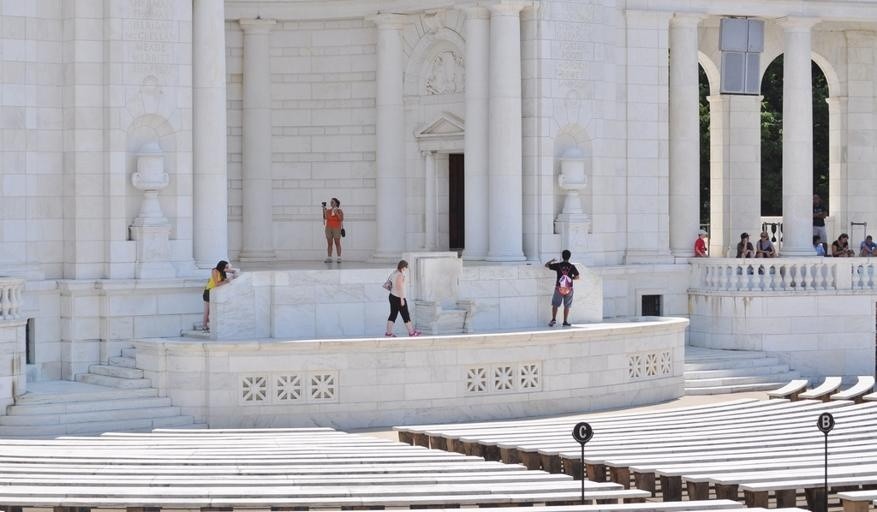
<box><xmin>736</xmin><ymin>232</ymin><xmax>755</xmax><ymax>274</ymax></box>
<box><xmin>384</xmin><ymin>260</ymin><xmax>421</xmax><ymax>337</ymax></box>
<box><xmin>859</xmin><ymin>236</ymin><xmax>877</xmax><ymax>257</ymax></box>
<box><xmin>323</xmin><ymin>197</ymin><xmax>344</xmax><ymax>263</ymax></box>
<box><xmin>546</xmin><ymin>249</ymin><xmax>579</xmax><ymax>327</ymax></box>
<box><xmin>201</xmin><ymin>261</ymin><xmax>230</xmax><ymax>333</ymax></box>
<box><xmin>832</xmin><ymin>233</ymin><xmax>853</xmax><ymax>256</ymax></box>
<box><xmin>813</xmin><ymin>194</ymin><xmax>827</xmax><ymax>243</ymax></box>
<box><xmin>813</xmin><ymin>236</ymin><xmax>825</xmax><ymax>256</ymax></box>
<box><xmin>694</xmin><ymin>230</ymin><xmax>708</xmax><ymax>256</ymax></box>
<box><xmin>755</xmin><ymin>232</ymin><xmax>776</xmax><ymax>274</ymax></box>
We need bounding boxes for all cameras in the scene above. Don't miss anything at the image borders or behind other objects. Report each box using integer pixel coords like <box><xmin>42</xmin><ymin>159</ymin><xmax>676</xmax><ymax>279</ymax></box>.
<box><xmin>322</xmin><ymin>201</ymin><xmax>326</xmax><ymax>206</ymax></box>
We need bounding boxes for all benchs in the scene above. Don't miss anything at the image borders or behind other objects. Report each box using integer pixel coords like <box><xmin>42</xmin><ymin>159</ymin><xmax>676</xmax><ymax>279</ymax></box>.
<box><xmin>1</xmin><ymin>375</ymin><xmax>877</xmax><ymax>511</ymax></box>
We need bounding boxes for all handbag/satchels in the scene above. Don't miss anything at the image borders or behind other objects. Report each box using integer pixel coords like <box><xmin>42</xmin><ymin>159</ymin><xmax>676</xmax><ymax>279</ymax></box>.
<box><xmin>341</xmin><ymin>229</ymin><xmax>345</xmax><ymax>237</ymax></box>
<box><xmin>383</xmin><ymin>280</ymin><xmax>392</xmax><ymax>290</ymax></box>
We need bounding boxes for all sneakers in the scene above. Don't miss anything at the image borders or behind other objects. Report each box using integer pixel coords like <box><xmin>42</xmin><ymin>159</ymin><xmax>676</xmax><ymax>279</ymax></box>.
<box><xmin>324</xmin><ymin>258</ymin><xmax>341</xmax><ymax>263</ymax></box>
<box><xmin>385</xmin><ymin>332</ymin><xmax>395</xmax><ymax>337</ymax></box>
<box><xmin>409</xmin><ymin>331</ymin><xmax>421</xmax><ymax>336</ymax></box>
<box><xmin>549</xmin><ymin>319</ymin><xmax>570</xmax><ymax>326</ymax></box>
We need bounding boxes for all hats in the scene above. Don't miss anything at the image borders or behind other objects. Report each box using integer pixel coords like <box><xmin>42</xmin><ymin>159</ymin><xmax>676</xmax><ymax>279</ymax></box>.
<box><xmin>741</xmin><ymin>233</ymin><xmax>748</xmax><ymax>238</ymax></box>
<box><xmin>761</xmin><ymin>232</ymin><xmax>768</xmax><ymax>237</ymax></box>
<box><xmin>699</xmin><ymin>230</ymin><xmax>707</xmax><ymax>234</ymax></box>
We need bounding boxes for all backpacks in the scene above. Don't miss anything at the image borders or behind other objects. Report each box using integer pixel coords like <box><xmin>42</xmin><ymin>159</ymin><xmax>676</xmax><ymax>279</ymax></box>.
<box><xmin>558</xmin><ymin>265</ymin><xmax>573</xmax><ymax>296</ymax></box>
